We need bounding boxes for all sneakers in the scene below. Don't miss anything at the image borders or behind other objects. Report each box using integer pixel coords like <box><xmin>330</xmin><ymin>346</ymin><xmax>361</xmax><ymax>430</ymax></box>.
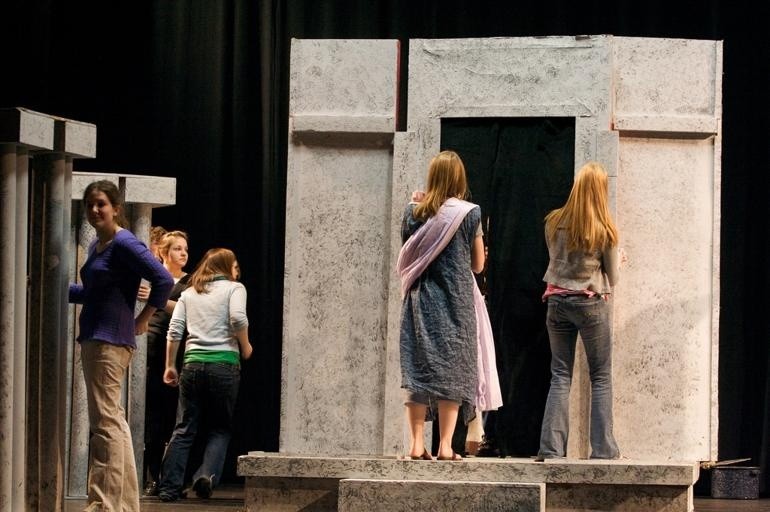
<box><xmin>144</xmin><ymin>475</ymin><xmax>214</xmax><ymax>503</ymax></box>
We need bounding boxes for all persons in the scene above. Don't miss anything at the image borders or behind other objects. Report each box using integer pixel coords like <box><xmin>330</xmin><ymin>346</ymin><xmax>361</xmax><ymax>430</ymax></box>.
<box><xmin>396</xmin><ymin>149</ymin><xmax>506</xmax><ymax>461</ymax></box>
<box><xmin>536</xmin><ymin>161</ymin><xmax>630</xmax><ymax>461</ymax></box>
<box><xmin>68</xmin><ymin>179</ymin><xmax>176</xmax><ymax>512</ymax></box>
<box><xmin>144</xmin><ymin>226</ymin><xmax>169</xmax><ymax>262</ymax></box>
<box><xmin>159</xmin><ymin>246</ymin><xmax>254</xmax><ymax>502</ymax></box>
<box><xmin>136</xmin><ymin>228</ymin><xmax>196</xmax><ymax>499</ymax></box>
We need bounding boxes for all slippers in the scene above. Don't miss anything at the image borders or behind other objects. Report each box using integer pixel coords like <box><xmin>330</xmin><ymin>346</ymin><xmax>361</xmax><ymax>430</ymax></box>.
<box><xmin>438</xmin><ymin>451</ymin><xmax>465</xmax><ymax>461</ymax></box>
<box><xmin>410</xmin><ymin>446</ymin><xmax>435</xmax><ymax>460</ymax></box>
